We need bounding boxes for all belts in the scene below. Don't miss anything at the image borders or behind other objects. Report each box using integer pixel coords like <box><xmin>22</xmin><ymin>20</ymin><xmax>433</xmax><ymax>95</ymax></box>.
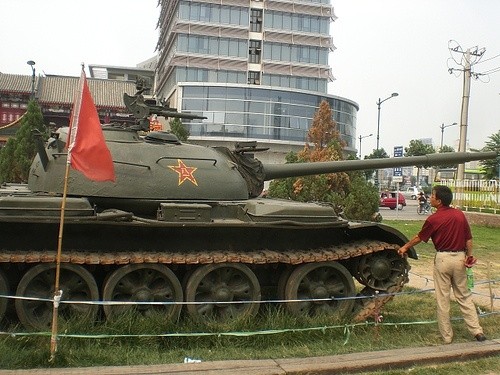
<box><xmin>437</xmin><ymin>250</ymin><xmax>466</xmax><ymax>252</ymax></box>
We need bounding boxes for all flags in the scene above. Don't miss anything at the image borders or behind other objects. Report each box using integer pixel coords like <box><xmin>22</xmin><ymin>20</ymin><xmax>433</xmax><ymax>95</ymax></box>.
<box><xmin>66</xmin><ymin>69</ymin><xmax>116</xmax><ymax>184</ymax></box>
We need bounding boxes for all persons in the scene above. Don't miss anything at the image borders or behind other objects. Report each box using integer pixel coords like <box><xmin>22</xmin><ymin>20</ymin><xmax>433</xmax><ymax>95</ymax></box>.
<box><xmin>418</xmin><ymin>192</ymin><xmax>428</xmax><ymax>214</ymax></box>
<box><xmin>398</xmin><ymin>184</ymin><xmax>487</xmax><ymax>343</ymax></box>
<box><xmin>392</xmin><ymin>186</ymin><xmax>395</xmax><ymax>191</ymax></box>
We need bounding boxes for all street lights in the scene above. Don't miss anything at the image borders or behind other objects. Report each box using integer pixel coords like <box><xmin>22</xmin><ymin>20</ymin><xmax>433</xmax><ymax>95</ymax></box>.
<box><xmin>440</xmin><ymin>121</ymin><xmax>458</xmax><ymax>154</ymax></box>
<box><xmin>375</xmin><ymin>92</ymin><xmax>400</xmax><ymax>198</ymax></box>
<box><xmin>357</xmin><ymin>133</ymin><xmax>373</xmax><ymax>161</ymax></box>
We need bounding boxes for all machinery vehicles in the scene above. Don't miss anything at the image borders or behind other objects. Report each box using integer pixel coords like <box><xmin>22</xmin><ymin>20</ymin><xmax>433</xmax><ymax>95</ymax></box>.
<box><xmin>0</xmin><ymin>73</ymin><xmax>498</xmax><ymax>336</ymax></box>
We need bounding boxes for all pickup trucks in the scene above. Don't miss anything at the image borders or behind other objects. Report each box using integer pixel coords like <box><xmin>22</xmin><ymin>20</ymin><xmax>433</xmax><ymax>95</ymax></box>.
<box><xmin>377</xmin><ymin>191</ymin><xmax>407</xmax><ymax>210</ymax></box>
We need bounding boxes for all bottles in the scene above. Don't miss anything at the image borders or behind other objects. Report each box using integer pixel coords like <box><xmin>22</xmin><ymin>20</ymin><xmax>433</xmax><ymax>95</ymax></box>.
<box><xmin>467</xmin><ymin>267</ymin><xmax>474</xmax><ymax>289</ymax></box>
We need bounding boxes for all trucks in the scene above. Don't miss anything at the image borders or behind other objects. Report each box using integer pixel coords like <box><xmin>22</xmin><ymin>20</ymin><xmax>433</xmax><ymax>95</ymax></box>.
<box><xmin>399</xmin><ymin>186</ymin><xmax>418</xmax><ymax>200</ymax></box>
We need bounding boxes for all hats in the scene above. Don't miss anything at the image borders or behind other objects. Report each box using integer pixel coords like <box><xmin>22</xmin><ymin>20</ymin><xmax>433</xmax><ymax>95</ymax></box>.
<box><xmin>420</xmin><ymin>192</ymin><xmax>424</xmax><ymax>195</ymax></box>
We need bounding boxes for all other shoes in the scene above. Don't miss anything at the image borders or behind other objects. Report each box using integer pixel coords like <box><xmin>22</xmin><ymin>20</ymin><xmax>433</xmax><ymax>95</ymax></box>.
<box><xmin>427</xmin><ymin>210</ymin><xmax>429</xmax><ymax>211</ymax></box>
<box><xmin>476</xmin><ymin>333</ymin><xmax>486</xmax><ymax>341</ymax></box>
<box><xmin>419</xmin><ymin>212</ymin><xmax>421</xmax><ymax>213</ymax></box>
<box><xmin>446</xmin><ymin>329</ymin><xmax>455</xmax><ymax>344</ymax></box>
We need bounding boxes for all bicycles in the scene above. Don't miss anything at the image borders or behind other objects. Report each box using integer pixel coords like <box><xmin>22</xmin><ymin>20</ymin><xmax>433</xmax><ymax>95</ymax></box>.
<box><xmin>417</xmin><ymin>196</ymin><xmax>438</xmax><ymax>216</ymax></box>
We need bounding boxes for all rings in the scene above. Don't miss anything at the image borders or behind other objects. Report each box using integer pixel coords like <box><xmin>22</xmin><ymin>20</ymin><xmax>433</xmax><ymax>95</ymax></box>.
<box><xmin>398</xmin><ymin>250</ymin><xmax>400</xmax><ymax>253</ymax></box>
